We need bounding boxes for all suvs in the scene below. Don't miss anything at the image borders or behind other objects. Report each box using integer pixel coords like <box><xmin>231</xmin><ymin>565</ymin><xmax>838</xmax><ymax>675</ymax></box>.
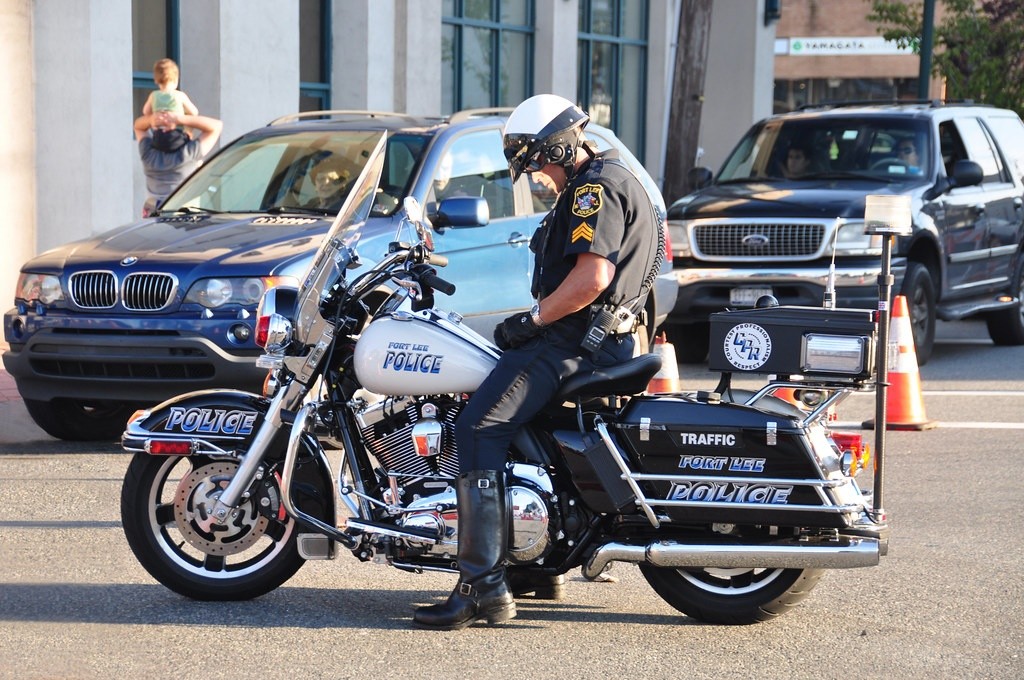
<box><xmin>1</xmin><ymin>107</ymin><xmax>679</xmax><ymax>440</ymax></box>
<box><xmin>663</xmin><ymin>98</ymin><xmax>1024</xmax><ymax>367</ymax></box>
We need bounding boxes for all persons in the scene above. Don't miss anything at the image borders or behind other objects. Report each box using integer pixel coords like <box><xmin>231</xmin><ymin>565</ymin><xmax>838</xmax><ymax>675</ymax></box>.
<box><xmin>300</xmin><ymin>158</ymin><xmax>350</xmax><ymax>210</ymax></box>
<box><xmin>788</xmin><ymin>148</ymin><xmax>811</xmax><ymax>174</ymax></box>
<box><xmin>135</xmin><ymin>58</ymin><xmax>223</xmax><ymax>209</ymax></box>
<box><xmin>896</xmin><ymin>136</ymin><xmax>921</xmax><ymax>164</ymax></box>
<box><xmin>412</xmin><ymin>94</ymin><xmax>665</xmax><ymax>630</ymax></box>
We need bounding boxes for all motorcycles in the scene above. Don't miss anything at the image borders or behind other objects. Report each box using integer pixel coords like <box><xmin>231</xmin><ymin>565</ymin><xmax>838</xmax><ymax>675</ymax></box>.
<box><xmin>119</xmin><ymin>128</ymin><xmax>913</xmax><ymax>626</ymax></box>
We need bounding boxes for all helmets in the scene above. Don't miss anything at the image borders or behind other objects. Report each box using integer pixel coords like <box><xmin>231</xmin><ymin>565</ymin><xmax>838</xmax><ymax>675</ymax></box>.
<box><xmin>503</xmin><ymin>94</ymin><xmax>589</xmax><ymax>183</ymax></box>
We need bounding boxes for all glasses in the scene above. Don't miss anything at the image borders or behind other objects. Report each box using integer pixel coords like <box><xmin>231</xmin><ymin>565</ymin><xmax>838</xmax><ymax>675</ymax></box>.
<box><xmin>895</xmin><ymin>147</ymin><xmax>911</xmax><ymax>155</ymax></box>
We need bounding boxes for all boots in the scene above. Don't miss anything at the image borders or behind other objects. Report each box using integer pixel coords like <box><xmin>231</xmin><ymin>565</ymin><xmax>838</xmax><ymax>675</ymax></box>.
<box><xmin>412</xmin><ymin>469</ymin><xmax>516</xmax><ymax>630</ymax></box>
<box><xmin>504</xmin><ymin>551</ymin><xmax>565</xmax><ymax>599</ymax></box>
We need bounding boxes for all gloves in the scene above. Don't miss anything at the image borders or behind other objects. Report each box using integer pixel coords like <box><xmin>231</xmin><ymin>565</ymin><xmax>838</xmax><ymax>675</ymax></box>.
<box><xmin>494</xmin><ymin>311</ymin><xmax>540</xmax><ymax>351</ymax></box>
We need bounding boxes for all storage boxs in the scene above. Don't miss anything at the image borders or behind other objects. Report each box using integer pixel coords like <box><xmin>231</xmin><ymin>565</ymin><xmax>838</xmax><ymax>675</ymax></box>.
<box><xmin>707</xmin><ymin>304</ymin><xmax>877</xmax><ymax>379</ymax></box>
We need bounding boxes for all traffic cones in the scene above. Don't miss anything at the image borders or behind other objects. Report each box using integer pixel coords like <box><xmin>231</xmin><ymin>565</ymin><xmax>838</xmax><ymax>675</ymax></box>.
<box><xmin>646</xmin><ymin>331</ymin><xmax>679</xmax><ymax>393</ymax></box>
<box><xmin>861</xmin><ymin>295</ymin><xmax>939</xmax><ymax>432</ymax></box>
